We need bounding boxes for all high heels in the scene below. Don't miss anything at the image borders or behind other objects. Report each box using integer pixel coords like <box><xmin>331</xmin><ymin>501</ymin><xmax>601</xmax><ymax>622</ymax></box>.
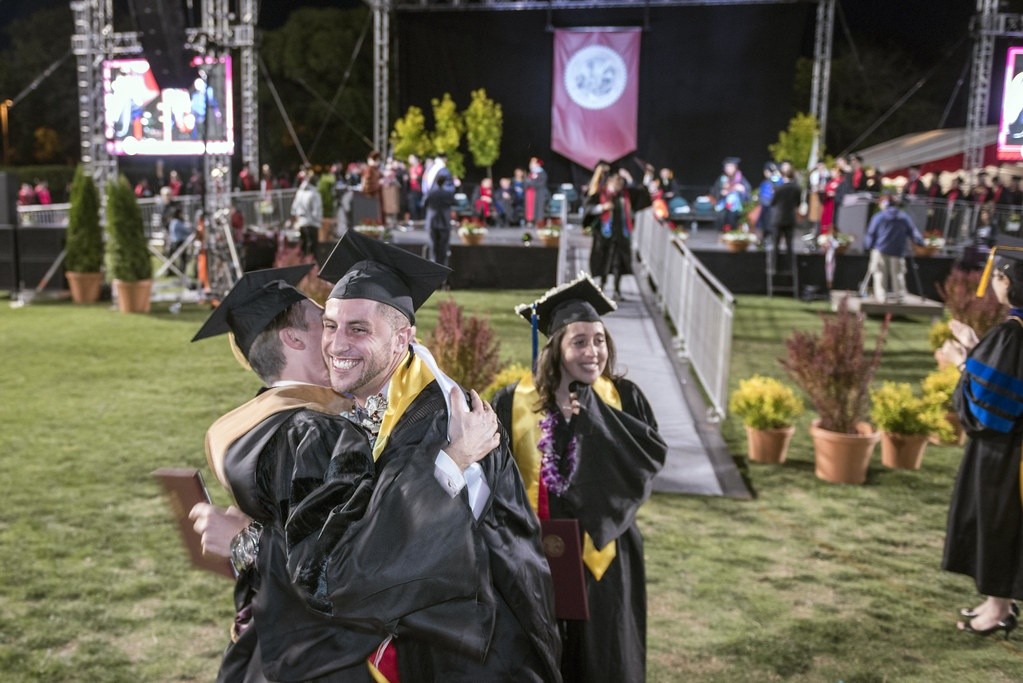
<box><xmin>956</xmin><ymin>611</ymin><xmax>1017</xmax><ymax>641</ymax></box>
<box><xmin>960</xmin><ymin>602</ymin><xmax>1020</xmax><ymax>622</ymax></box>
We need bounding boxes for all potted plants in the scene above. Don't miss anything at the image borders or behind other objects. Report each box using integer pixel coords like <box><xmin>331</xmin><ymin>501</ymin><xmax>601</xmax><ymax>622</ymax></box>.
<box><xmin>457</xmin><ymin>222</ymin><xmax>487</xmax><ymax>246</ymax></box>
<box><xmin>915</xmin><ymin>234</ymin><xmax>938</xmax><ymax>257</ymax></box>
<box><xmin>832</xmin><ymin>234</ymin><xmax>850</xmax><ymax>252</ymax></box>
<box><xmin>721</xmin><ymin>230</ymin><xmax>748</xmax><ymax>252</ymax></box>
<box><xmin>104</xmin><ymin>172</ymin><xmax>155</xmax><ymax>313</ymax></box>
<box><xmin>871</xmin><ymin>362</ymin><xmax>971</xmax><ymax>472</ymax></box>
<box><xmin>730</xmin><ymin>373</ymin><xmax>802</xmax><ymax>461</ymax></box>
<box><xmin>782</xmin><ymin>295</ymin><xmax>892</xmax><ymax>487</ymax></box>
<box><xmin>536</xmin><ymin>225</ymin><xmax>561</xmax><ymax>249</ymax></box>
<box><xmin>64</xmin><ymin>162</ymin><xmax>105</xmax><ymax>304</ymax></box>
<box><xmin>352</xmin><ymin>224</ymin><xmax>386</xmax><ymax>240</ymax></box>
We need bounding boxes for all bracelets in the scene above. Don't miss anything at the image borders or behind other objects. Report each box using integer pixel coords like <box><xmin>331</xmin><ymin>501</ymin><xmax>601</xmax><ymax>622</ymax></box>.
<box><xmin>959</xmin><ymin>361</ymin><xmax>966</xmax><ymax>370</ymax></box>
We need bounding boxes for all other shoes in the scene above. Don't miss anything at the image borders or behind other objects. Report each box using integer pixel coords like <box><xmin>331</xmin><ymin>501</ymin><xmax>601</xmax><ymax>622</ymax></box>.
<box><xmin>612</xmin><ymin>293</ymin><xmax>624</xmax><ymax>301</ymax></box>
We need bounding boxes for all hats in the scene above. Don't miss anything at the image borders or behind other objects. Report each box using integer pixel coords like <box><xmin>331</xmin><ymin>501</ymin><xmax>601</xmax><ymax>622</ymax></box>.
<box><xmin>513</xmin><ymin>270</ymin><xmax>617</xmax><ymax>376</ymax></box>
<box><xmin>975</xmin><ymin>236</ymin><xmax>1023</xmax><ymax>298</ymax></box>
<box><xmin>317</xmin><ymin>227</ymin><xmax>455</xmax><ymax>328</ymax></box>
<box><xmin>191</xmin><ymin>263</ymin><xmax>316</xmax><ymax>371</ymax></box>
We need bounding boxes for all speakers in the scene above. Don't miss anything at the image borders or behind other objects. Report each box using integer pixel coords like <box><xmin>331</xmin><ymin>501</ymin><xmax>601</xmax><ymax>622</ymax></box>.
<box><xmin>129</xmin><ymin>0</ymin><xmax>196</xmax><ymax>89</ymax></box>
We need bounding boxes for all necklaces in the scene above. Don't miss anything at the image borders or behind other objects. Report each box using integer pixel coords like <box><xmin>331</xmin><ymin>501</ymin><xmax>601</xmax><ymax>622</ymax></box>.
<box><xmin>536</xmin><ymin>397</ymin><xmax>582</xmax><ymax>498</ymax></box>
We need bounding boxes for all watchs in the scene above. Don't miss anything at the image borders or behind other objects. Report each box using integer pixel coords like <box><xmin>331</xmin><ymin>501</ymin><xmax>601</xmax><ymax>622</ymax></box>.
<box><xmin>231</xmin><ymin>522</ymin><xmax>264</xmax><ymax>574</ymax></box>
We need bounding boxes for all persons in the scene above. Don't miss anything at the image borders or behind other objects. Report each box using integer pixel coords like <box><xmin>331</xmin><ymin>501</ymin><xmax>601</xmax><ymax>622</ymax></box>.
<box><xmin>110</xmin><ymin>68</ymin><xmax>217</xmax><ymax>142</ymax></box>
<box><xmin>190</xmin><ymin>229</ymin><xmax>564</xmax><ymax>683</ymax></box>
<box><xmin>713</xmin><ymin>155</ymin><xmax>1023</xmax><ymax>300</ymax></box>
<box><xmin>130</xmin><ymin>151</ymin><xmax>547</xmax><ymax>277</ymax></box>
<box><xmin>934</xmin><ymin>230</ymin><xmax>1023</xmax><ymax>640</ymax></box>
<box><xmin>17</xmin><ymin>179</ymin><xmax>53</xmax><ymax>223</ymax></box>
<box><xmin>583</xmin><ymin>161</ymin><xmax>680</xmax><ymax>302</ymax></box>
<box><xmin>488</xmin><ymin>275</ymin><xmax>668</xmax><ymax>683</ymax></box>
